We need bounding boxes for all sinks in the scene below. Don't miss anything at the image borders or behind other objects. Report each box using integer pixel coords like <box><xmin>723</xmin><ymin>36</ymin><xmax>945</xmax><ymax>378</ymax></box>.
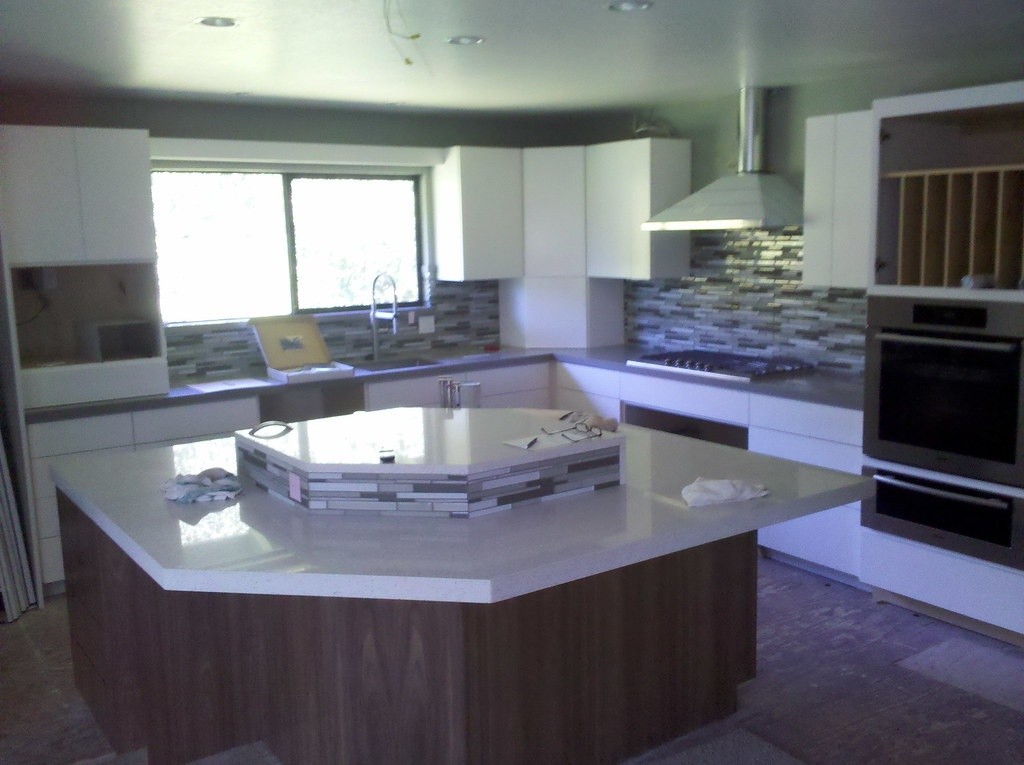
<box><xmin>349</xmin><ymin>356</ymin><xmax>442</xmax><ymax>372</ymax></box>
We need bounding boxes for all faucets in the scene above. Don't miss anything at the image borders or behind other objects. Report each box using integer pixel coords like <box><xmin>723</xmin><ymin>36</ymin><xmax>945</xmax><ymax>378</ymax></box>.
<box><xmin>370</xmin><ymin>273</ymin><xmax>398</xmax><ymax>360</ymax></box>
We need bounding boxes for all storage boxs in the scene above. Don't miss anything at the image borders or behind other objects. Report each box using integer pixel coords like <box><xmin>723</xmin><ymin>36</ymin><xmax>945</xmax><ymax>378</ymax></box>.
<box><xmin>589</xmin><ymin>137</ymin><xmax>692</xmax><ymax>280</ymax></box>
<box><xmin>522</xmin><ymin>146</ymin><xmax>587</xmax><ymax>278</ymax></box>
<box><xmin>431</xmin><ymin>137</ymin><xmax>523</xmax><ymax>282</ymax></box>
<box><xmin>247</xmin><ymin>314</ymin><xmax>355</xmax><ymax>384</ymax></box>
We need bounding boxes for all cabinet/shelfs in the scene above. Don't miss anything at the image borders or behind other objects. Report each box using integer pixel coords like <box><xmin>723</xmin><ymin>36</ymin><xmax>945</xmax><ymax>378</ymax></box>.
<box><xmin>24</xmin><ymin>398</ymin><xmax>136</xmax><ymax>599</ymax></box>
<box><xmin>133</xmin><ymin>374</ymin><xmax>261</xmax><ymax>449</ymax></box>
<box><xmin>426</xmin><ymin>343</ymin><xmax>551</xmax><ymax>408</ymax></box>
<box><xmin>0</xmin><ymin>123</ymin><xmax>157</xmax><ymax>266</ymax></box>
<box><xmin>748</xmin><ymin>371</ymin><xmax>864</xmax><ymax>589</ymax></box>
<box><xmin>867</xmin><ymin>80</ymin><xmax>1024</xmax><ymax>304</ymax></box>
<box><xmin>550</xmin><ymin>345</ymin><xmax>692</xmax><ymax>433</ymax></box>
<box><xmin>800</xmin><ymin>110</ymin><xmax>870</xmax><ymax>287</ymax></box>
